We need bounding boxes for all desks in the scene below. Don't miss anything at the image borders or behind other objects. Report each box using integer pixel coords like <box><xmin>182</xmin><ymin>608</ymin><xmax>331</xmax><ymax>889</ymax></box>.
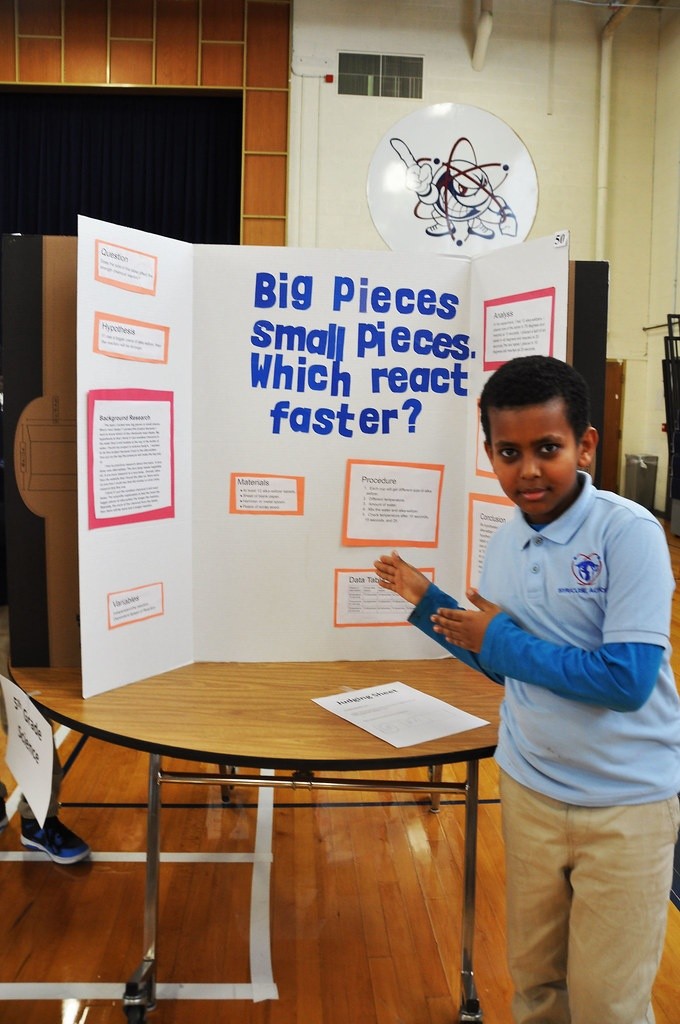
<box><xmin>0</xmin><ymin>663</ymin><xmax>502</xmax><ymax>1024</ymax></box>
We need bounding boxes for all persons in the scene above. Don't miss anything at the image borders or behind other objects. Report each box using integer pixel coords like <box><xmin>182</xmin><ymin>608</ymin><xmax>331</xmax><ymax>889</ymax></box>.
<box><xmin>0</xmin><ymin>604</ymin><xmax>89</xmax><ymax>866</ymax></box>
<box><xmin>373</xmin><ymin>355</ymin><xmax>680</xmax><ymax>1024</ymax></box>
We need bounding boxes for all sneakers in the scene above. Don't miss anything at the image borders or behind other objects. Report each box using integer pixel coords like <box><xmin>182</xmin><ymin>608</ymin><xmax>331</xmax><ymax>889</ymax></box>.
<box><xmin>0</xmin><ymin>798</ymin><xmax>8</xmax><ymax>827</ymax></box>
<box><xmin>20</xmin><ymin>815</ymin><xmax>89</xmax><ymax>864</ymax></box>
